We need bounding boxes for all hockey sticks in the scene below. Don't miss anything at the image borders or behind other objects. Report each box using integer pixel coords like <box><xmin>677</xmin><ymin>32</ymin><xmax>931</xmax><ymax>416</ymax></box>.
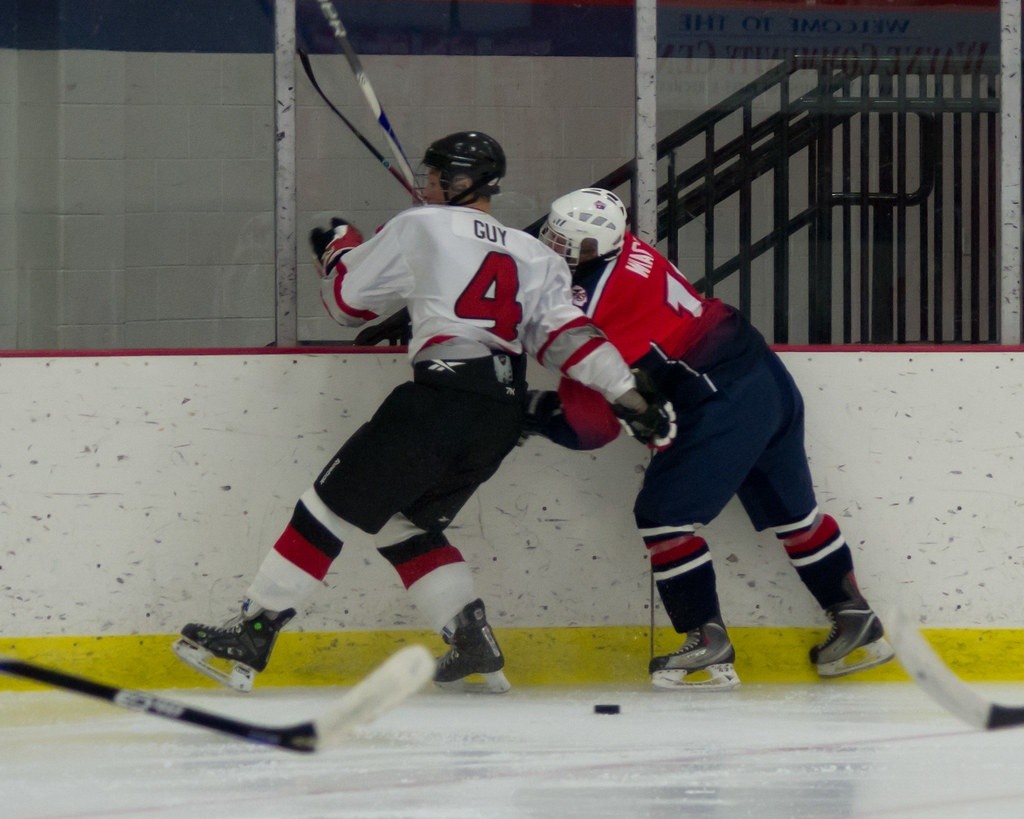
<box><xmin>319</xmin><ymin>0</ymin><xmax>428</xmax><ymax>207</ymax></box>
<box><xmin>0</xmin><ymin>641</ymin><xmax>439</xmax><ymax>754</ymax></box>
<box><xmin>294</xmin><ymin>24</ymin><xmax>427</xmax><ymax>205</ymax></box>
<box><xmin>882</xmin><ymin>605</ymin><xmax>1024</xmax><ymax>732</ymax></box>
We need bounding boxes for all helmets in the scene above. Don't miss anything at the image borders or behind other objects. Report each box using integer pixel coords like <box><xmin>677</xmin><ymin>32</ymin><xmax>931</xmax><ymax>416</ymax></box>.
<box><xmin>548</xmin><ymin>187</ymin><xmax>627</xmax><ymax>261</ymax></box>
<box><xmin>424</xmin><ymin>131</ymin><xmax>506</xmax><ymax>194</ymax></box>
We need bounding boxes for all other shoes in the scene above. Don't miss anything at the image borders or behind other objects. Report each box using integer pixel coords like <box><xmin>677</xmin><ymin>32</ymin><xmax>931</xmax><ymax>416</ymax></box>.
<box><xmin>648</xmin><ymin>615</ymin><xmax>742</xmax><ymax>689</ymax></box>
<box><xmin>809</xmin><ymin>598</ymin><xmax>895</xmax><ymax>677</ymax></box>
<box><xmin>432</xmin><ymin>599</ymin><xmax>511</xmax><ymax>694</ymax></box>
<box><xmin>173</xmin><ymin>606</ymin><xmax>297</xmax><ymax>693</ymax></box>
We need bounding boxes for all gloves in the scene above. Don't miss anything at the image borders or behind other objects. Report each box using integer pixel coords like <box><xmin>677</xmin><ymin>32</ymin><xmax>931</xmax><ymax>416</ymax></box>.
<box><xmin>310</xmin><ymin>217</ymin><xmax>364</xmax><ymax>276</ymax></box>
<box><xmin>615</xmin><ymin>398</ymin><xmax>679</xmax><ymax>452</ymax></box>
<box><xmin>517</xmin><ymin>387</ymin><xmax>556</xmax><ymax>448</ymax></box>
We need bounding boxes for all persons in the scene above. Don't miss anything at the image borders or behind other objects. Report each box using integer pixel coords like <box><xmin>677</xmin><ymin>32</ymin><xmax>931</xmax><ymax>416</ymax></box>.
<box><xmin>181</xmin><ymin>129</ymin><xmax>674</xmax><ymax>683</ymax></box>
<box><xmin>525</xmin><ymin>187</ymin><xmax>891</xmax><ymax>672</ymax></box>
